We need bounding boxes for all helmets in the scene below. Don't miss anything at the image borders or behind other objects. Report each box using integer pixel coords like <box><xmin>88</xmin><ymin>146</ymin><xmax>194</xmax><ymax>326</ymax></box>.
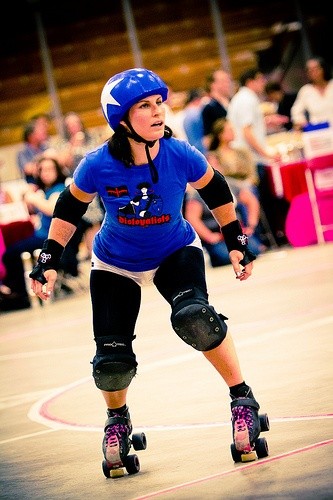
<box><xmin>101</xmin><ymin>68</ymin><xmax>168</xmax><ymax>132</ymax></box>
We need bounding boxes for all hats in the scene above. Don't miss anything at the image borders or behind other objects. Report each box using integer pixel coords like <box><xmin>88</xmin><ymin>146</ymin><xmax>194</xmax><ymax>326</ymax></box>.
<box><xmin>32</xmin><ymin>148</ymin><xmax>71</xmax><ymax>178</ymax></box>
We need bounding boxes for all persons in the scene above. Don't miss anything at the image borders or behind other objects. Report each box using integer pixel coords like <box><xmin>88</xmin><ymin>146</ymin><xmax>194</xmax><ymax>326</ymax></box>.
<box><xmin>225</xmin><ymin>70</ymin><xmax>279</xmax><ymax>248</ymax></box>
<box><xmin>29</xmin><ymin>69</ymin><xmax>268</xmax><ymax>478</ymax></box>
<box><xmin>185</xmin><ymin>155</ymin><xmax>264</xmax><ymax>267</ymax></box>
<box><xmin>2</xmin><ymin>158</ymin><xmax>75</xmax><ymax>299</ymax></box>
<box><xmin>17</xmin><ymin>67</ymin><xmax>301</xmax><ymax>181</ymax></box>
<box><xmin>290</xmin><ymin>59</ymin><xmax>333</xmax><ymax>132</ymax></box>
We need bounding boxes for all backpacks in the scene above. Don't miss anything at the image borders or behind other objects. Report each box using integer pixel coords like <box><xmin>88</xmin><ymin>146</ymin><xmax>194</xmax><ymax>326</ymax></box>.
<box><xmin>182</xmin><ymin>102</ymin><xmax>205</xmax><ymax>155</ymax></box>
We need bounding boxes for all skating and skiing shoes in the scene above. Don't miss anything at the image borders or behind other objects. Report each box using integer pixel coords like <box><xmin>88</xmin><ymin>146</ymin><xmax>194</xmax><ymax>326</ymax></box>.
<box><xmin>101</xmin><ymin>406</ymin><xmax>147</xmax><ymax>478</ymax></box>
<box><xmin>231</xmin><ymin>388</ymin><xmax>271</xmax><ymax>462</ymax></box>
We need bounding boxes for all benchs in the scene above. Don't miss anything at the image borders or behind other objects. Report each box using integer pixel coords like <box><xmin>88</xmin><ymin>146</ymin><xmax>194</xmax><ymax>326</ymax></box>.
<box><xmin>0</xmin><ymin>16</ymin><xmax>332</xmax><ymax>312</ymax></box>
